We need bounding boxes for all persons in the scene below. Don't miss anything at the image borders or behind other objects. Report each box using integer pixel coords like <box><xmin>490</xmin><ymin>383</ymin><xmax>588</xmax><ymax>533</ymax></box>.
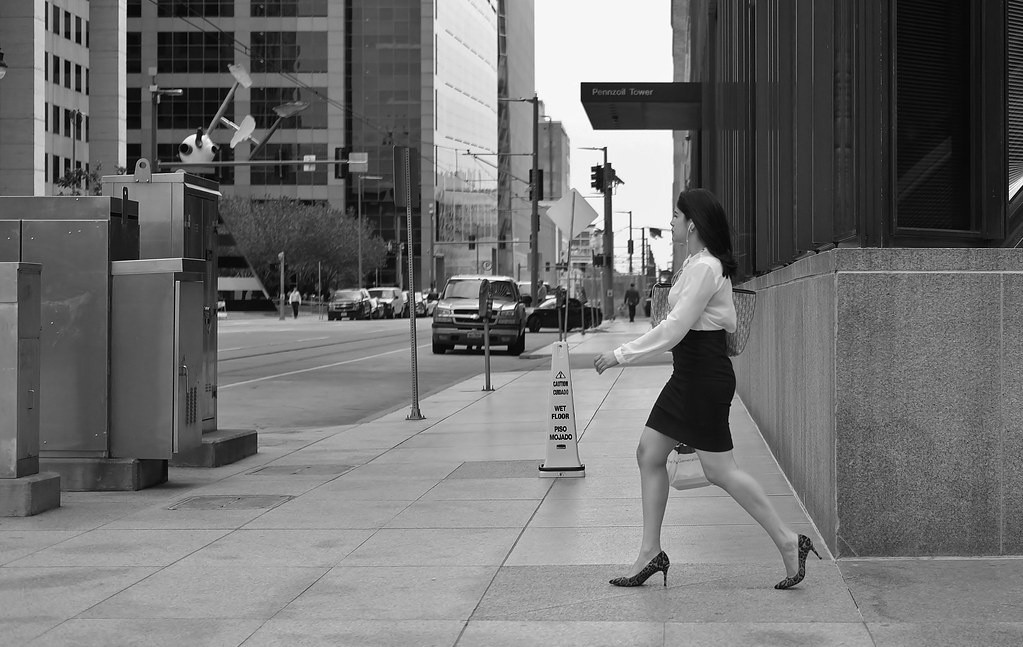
<box><xmin>537</xmin><ymin>279</ymin><xmax>547</xmax><ymax>302</ymax></box>
<box><xmin>289</xmin><ymin>287</ymin><xmax>301</xmax><ymax>319</ymax></box>
<box><xmin>624</xmin><ymin>283</ymin><xmax>640</xmax><ymax>321</ymax></box>
<box><xmin>592</xmin><ymin>186</ymin><xmax>823</xmax><ymax>586</ymax></box>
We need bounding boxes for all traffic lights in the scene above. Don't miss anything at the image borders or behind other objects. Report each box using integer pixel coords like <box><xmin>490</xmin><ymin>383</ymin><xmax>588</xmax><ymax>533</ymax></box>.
<box><xmin>591</xmin><ymin>165</ymin><xmax>603</xmax><ymax>188</ymax></box>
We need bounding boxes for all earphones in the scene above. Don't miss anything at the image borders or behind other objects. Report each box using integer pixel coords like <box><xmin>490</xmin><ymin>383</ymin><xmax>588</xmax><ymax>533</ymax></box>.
<box><xmin>688</xmin><ymin>222</ymin><xmax>693</xmax><ymax>230</ymax></box>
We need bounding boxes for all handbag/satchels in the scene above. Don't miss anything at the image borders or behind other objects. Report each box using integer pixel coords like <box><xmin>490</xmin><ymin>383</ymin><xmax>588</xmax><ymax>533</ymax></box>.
<box><xmin>649</xmin><ymin>283</ymin><xmax>756</xmax><ymax>356</ymax></box>
<box><xmin>668</xmin><ymin>442</ymin><xmax>712</xmax><ymax>491</ymax></box>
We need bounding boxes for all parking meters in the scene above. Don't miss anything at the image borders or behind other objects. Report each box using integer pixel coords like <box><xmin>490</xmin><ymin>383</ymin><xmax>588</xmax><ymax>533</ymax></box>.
<box><xmin>579</xmin><ymin>287</ymin><xmax>588</xmax><ymax>334</ymax></box>
<box><xmin>479</xmin><ymin>279</ymin><xmax>495</xmax><ymax>390</ymax></box>
<box><xmin>556</xmin><ymin>286</ymin><xmax>565</xmax><ymax>341</ymax></box>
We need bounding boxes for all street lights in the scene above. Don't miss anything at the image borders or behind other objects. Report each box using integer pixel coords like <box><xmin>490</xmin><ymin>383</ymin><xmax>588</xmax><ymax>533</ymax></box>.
<box><xmin>356</xmin><ymin>175</ymin><xmax>384</xmax><ymax>289</ymax></box>
<box><xmin>150</xmin><ymin>89</ymin><xmax>183</xmax><ymax>174</ymax></box>
<box><xmin>498</xmin><ymin>97</ymin><xmax>540</xmax><ymax>305</ymax></box>
<box><xmin>70</xmin><ymin>108</ymin><xmax>83</xmax><ymax>196</ymax></box>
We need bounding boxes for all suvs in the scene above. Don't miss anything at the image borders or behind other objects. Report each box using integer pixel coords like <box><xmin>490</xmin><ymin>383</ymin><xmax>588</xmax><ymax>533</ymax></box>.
<box><xmin>517</xmin><ymin>282</ymin><xmax>548</xmax><ymax>303</ymax></box>
<box><xmin>368</xmin><ymin>287</ymin><xmax>408</xmax><ymax>319</ymax></box>
<box><xmin>431</xmin><ymin>275</ymin><xmax>527</xmax><ymax>355</ymax></box>
<box><xmin>328</xmin><ymin>287</ymin><xmax>372</xmax><ymax>320</ymax></box>
<box><xmin>403</xmin><ymin>289</ymin><xmax>443</xmax><ymax>316</ymax></box>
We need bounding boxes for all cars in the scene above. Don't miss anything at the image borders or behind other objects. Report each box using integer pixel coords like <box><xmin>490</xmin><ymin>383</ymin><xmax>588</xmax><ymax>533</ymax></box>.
<box><xmin>545</xmin><ymin>295</ymin><xmax>555</xmax><ymax>301</ymax></box>
<box><xmin>525</xmin><ymin>297</ymin><xmax>601</xmax><ymax>332</ymax></box>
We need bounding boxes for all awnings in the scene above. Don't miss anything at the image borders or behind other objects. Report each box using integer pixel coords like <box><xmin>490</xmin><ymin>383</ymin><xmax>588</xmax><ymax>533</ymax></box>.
<box><xmin>580</xmin><ymin>81</ymin><xmax>701</xmax><ymax>129</ymax></box>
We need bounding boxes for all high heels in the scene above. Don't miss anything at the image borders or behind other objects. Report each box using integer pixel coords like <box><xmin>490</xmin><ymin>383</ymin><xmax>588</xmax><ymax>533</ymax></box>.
<box><xmin>608</xmin><ymin>551</ymin><xmax>670</xmax><ymax>587</ymax></box>
<box><xmin>774</xmin><ymin>533</ymin><xmax>822</xmax><ymax>589</ymax></box>
